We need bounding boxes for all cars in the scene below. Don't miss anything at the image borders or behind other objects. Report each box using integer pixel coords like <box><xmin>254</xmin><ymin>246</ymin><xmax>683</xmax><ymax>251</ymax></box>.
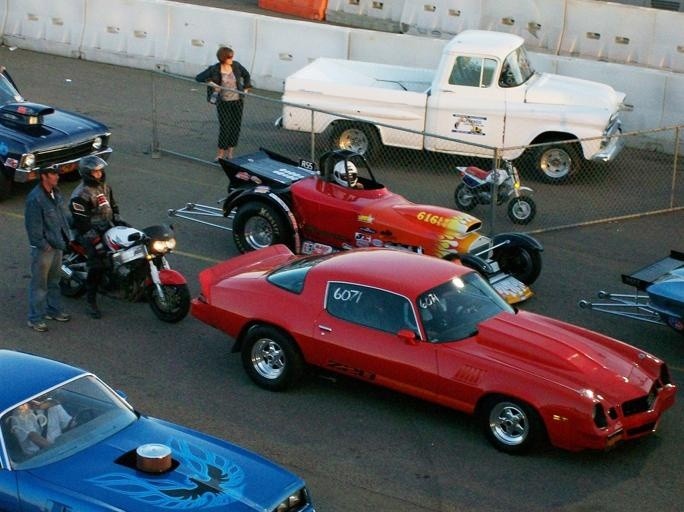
<box><xmin>2</xmin><ymin>60</ymin><xmax>113</xmax><ymax>178</ymax></box>
<box><xmin>1</xmin><ymin>347</ymin><xmax>309</xmax><ymax>506</ymax></box>
<box><xmin>592</xmin><ymin>239</ymin><xmax>682</xmax><ymax>336</ymax></box>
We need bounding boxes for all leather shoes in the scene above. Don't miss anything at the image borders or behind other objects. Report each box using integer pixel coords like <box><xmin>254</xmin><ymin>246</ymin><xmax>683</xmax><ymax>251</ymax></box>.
<box><xmin>85</xmin><ymin>303</ymin><xmax>101</xmax><ymax>317</ymax></box>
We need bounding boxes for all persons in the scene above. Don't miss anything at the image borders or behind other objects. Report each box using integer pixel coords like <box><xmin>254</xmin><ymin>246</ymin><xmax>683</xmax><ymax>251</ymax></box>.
<box><xmin>195</xmin><ymin>46</ymin><xmax>252</xmax><ymax>162</ymax></box>
<box><xmin>402</xmin><ymin>292</ymin><xmax>447</xmax><ymax>339</ymax></box>
<box><xmin>68</xmin><ymin>154</ymin><xmax>122</xmax><ymax>320</ymax></box>
<box><xmin>23</xmin><ymin>167</ymin><xmax>71</xmax><ymax>332</ymax></box>
<box><xmin>331</xmin><ymin>160</ymin><xmax>364</xmax><ymax>190</ymax></box>
<box><xmin>13</xmin><ymin>394</ymin><xmax>76</xmax><ymax>456</ymax></box>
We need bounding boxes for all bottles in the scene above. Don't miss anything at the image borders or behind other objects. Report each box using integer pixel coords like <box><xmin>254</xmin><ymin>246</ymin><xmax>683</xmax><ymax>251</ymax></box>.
<box><xmin>210</xmin><ymin>89</ymin><xmax>218</xmax><ymax>103</ymax></box>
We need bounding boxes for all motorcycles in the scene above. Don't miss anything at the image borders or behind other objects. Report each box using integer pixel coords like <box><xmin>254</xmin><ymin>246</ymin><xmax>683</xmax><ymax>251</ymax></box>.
<box><xmin>50</xmin><ymin>199</ymin><xmax>191</xmax><ymax>323</ymax></box>
<box><xmin>453</xmin><ymin>154</ymin><xmax>538</xmax><ymax>221</ymax></box>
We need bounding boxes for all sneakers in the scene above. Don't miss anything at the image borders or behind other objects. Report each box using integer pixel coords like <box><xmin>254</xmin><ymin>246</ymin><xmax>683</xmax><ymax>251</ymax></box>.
<box><xmin>45</xmin><ymin>307</ymin><xmax>70</xmax><ymax>322</ymax></box>
<box><xmin>27</xmin><ymin>320</ymin><xmax>48</xmax><ymax>332</ymax></box>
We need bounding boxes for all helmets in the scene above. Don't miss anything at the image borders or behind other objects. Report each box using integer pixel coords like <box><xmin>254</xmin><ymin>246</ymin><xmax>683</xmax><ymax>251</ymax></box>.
<box><xmin>78</xmin><ymin>156</ymin><xmax>108</xmax><ymax>185</ymax></box>
<box><xmin>333</xmin><ymin>160</ymin><xmax>358</xmax><ymax>187</ymax></box>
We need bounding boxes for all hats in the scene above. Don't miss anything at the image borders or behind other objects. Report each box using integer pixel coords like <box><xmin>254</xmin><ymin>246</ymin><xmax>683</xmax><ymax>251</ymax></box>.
<box><xmin>39</xmin><ymin>163</ymin><xmax>62</xmax><ymax>174</ymax></box>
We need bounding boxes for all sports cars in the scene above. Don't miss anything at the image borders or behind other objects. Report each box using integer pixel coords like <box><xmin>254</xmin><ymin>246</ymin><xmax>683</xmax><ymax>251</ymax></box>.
<box><xmin>197</xmin><ymin>234</ymin><xmax>676</xmax><ymax>457</ymax></box>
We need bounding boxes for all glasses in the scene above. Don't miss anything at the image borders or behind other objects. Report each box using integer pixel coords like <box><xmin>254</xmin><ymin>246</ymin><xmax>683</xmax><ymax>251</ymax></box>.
<box><xmin>45</xmin><ymin>164</ymin><xmax>60</xmax><ymax>170</ymax></box>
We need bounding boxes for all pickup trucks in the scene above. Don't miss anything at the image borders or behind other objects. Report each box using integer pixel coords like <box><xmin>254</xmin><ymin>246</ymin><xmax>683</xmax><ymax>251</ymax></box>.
<box><xmin>281</xmin><ymin>30</ymin><xmax>621</xmax><ymax>179</ymax></box>
